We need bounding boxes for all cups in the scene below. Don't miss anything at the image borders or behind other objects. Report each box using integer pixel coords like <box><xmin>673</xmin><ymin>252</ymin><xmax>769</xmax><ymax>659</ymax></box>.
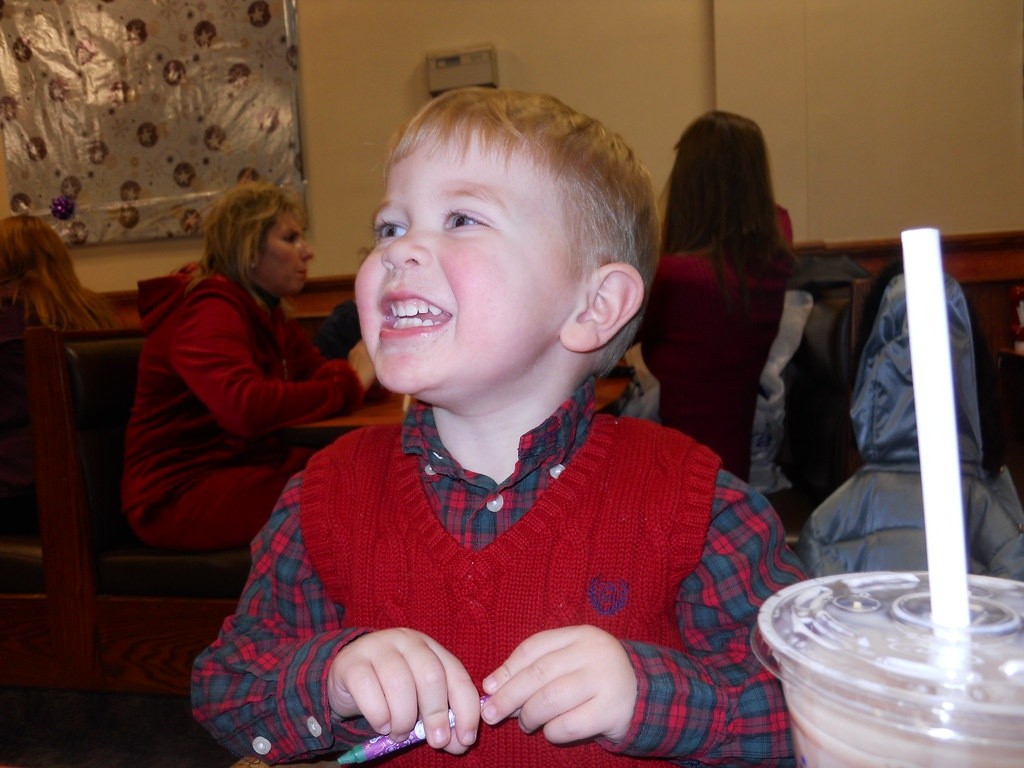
<box><xmin>750</xmin><ymin>568</ymin><xmax>1024</xmax><ymax>768</ymax></box>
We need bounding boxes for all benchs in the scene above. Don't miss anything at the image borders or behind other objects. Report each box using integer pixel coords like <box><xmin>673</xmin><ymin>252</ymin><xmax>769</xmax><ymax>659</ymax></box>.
<box><xmin>1</xmin><ymin>304</ymin><xmax>334</xmax><ymax>698</ymax></box>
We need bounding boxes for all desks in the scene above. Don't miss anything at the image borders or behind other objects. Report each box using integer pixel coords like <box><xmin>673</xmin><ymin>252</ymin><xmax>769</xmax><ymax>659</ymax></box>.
<box><xmin>271</xmin><ymin>365</ymin><xmax>632</xmax><ymax>453</ymax></box>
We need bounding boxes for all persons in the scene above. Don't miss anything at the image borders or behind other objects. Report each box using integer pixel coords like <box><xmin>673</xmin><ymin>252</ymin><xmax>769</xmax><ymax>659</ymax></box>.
<box><xmin>122</xmin><ymin>179</ymin><xmax>377</xmax><ymax>553</ymax></box>
<box><xmin>191</xmin><ymin>81</ymin><xmax>811</xmax><ymax>767</ymax></box>
<box><xmin>627</xmin><ymin>111</ymin><xmax>810</xmax><ymax>482</ymax></box>
<box><xmin>0</xmin><ymin>214</ymin><xmax>127</xmax><ymax>537</ymax></box>
